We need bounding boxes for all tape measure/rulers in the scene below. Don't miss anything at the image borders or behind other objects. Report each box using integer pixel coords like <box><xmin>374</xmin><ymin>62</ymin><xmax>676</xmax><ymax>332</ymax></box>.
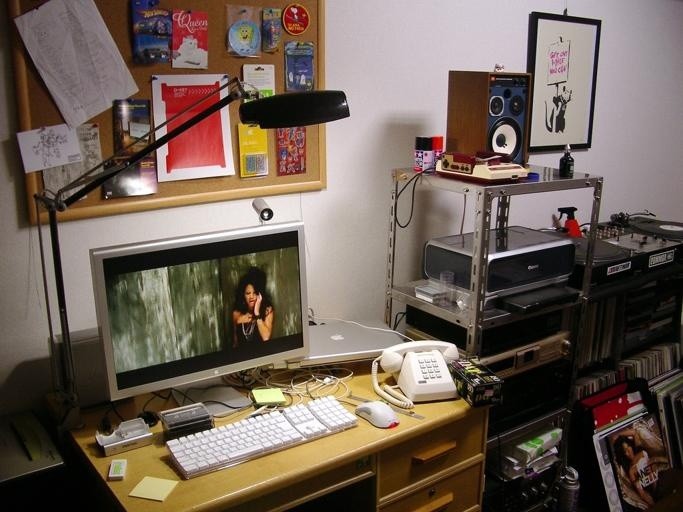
<box><xmin>348</xmin><ymin>394</ymin><xmax>425</xmax><ymax>420</ymax></box>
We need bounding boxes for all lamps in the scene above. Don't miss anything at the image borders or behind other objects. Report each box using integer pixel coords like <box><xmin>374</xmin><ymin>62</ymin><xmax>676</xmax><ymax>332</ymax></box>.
<box><xmin>28</xmin><ymin>72</ymin><xmax>349</xmax><ymax>434</ymax></box>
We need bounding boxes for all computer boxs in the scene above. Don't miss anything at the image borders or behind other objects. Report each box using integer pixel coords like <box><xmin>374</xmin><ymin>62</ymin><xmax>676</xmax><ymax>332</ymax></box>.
<box><xmin>423</xmin><ymin>222</ymin><xmax>578</xmax><ymax>295</ymax></box>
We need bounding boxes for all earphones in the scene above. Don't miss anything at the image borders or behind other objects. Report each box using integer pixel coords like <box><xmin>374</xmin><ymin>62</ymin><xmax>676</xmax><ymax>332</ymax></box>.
<box><xmin>323</xmin><ymin>377</ymin><xmax>332</xmax><ymax>385</ymax></box>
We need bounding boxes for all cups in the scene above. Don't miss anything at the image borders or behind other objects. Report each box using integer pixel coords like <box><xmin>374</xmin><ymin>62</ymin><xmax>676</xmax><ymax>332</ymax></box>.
<box><xmin>439</xmin><ymin>270</ymin><xmax>454</xmax><ymax>306</ymax></box>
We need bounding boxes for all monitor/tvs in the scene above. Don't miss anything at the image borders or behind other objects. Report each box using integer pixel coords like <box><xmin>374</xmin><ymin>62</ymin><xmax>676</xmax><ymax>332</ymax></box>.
<box><xmin>85</xmin><ymin>219</ymin><xmax>310</xmax><ymax>420</ymax></box>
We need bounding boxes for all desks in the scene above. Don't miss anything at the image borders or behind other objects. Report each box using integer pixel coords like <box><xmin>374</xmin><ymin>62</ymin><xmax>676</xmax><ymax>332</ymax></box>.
<box><xmin>58</xmin><ymin>360</ymin><xmax>489</xmax><ymax>511</ymax></box>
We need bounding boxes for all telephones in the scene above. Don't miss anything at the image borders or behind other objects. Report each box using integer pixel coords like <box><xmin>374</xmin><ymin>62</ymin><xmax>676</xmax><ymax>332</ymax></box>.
<box><xmin>379</xmin><ymin>340</ymin><xmax>459</xmax><ymax>403</ymax></box>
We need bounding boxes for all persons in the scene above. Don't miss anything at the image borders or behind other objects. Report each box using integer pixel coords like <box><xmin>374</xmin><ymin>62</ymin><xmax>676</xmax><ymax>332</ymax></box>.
<box><xmin>228</xmin><ymin>271</ymin><xmax>274</xmax><ymax>350</ymax></box>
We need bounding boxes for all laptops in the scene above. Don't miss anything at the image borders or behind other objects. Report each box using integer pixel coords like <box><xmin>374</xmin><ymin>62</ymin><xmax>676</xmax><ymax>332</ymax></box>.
<box><xmin>266</xmin><ymin>317</ymin><xmax>407</xmax><ymax>373</ymax></box>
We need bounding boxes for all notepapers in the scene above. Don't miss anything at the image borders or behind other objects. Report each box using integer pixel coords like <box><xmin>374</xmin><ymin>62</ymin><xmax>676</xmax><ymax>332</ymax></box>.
<box><xmin>251</xmin><ymin>388</ymin><xmax>286</xmax><ymax>406</ymax></box>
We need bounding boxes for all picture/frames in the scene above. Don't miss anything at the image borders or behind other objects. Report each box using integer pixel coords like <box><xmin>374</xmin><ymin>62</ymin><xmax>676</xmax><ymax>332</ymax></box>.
<box><xmin>529</xmin><ymin>10</ymin><xmax>602</xmax><ymax>153</ymax></box>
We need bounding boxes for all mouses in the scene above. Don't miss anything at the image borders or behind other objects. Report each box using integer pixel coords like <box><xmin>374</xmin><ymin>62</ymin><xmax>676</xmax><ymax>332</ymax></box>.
<box><xmin>353</xmin><ymin>400</ymin><xmax>401</xmax><ymax>431</ymax></box>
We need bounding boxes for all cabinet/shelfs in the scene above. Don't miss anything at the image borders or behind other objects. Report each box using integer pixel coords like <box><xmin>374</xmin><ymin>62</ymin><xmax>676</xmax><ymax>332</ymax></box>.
<box><xmin>384</xmin><ymin>161</ymin><xmax>680</xmax><ymax>512</ymax></box>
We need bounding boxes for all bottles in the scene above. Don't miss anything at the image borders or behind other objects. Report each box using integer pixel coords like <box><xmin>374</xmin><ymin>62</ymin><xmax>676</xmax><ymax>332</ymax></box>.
<box><xmin>559</xmin><ymin>143</ymin><xmax>575</xmax><ymax>179</ymax></box>
<box><xmin>413</xmin><ymin>134</ymin><xmax>443</xmax><ymax>174</ymax></box>
<box><xmin>560</xmin><ymin>466</ymin><xmax>580</xmax><ymax>512</ymax></box>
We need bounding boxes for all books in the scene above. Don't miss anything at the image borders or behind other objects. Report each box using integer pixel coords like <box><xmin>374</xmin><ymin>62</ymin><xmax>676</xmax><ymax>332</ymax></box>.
<box><xmin>568</xmin><ymin>271</ymin><xmax>681</xmax><ymax>511</ymax></box>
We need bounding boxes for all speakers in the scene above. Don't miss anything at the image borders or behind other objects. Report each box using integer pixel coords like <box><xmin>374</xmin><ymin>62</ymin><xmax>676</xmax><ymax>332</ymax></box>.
<box><xmin>444</xmin><ymin>69</ymin><xmax>532</xmax><ymax>167</ymax></box>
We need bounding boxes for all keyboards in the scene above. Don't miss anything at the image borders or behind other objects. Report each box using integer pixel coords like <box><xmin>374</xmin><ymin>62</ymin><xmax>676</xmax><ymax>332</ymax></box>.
<box><xmin>166</xmin><ymin>394</ymin><xmax>359</xmax><ymax>480</ymax></box>
